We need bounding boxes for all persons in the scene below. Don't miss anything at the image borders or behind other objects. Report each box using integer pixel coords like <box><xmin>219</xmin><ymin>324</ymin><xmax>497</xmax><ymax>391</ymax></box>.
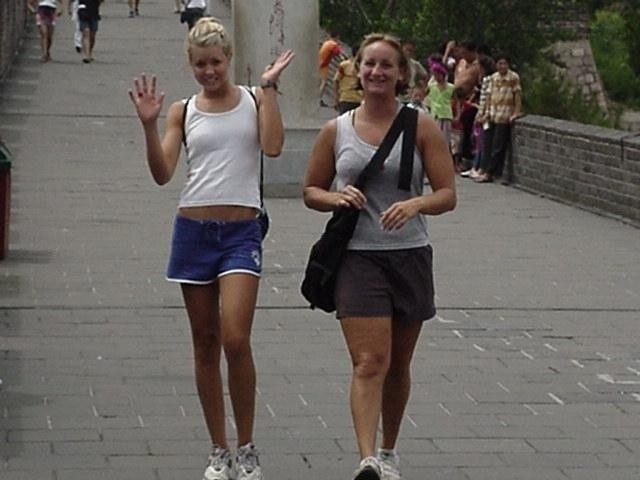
<box><xmin>302</xmin><ymin>32</ymin><xmax>458</xmax><ymax>480</ymax></box>
<box><xmin>26</xmin><ymin>0</ymin><xmax>210</xmax><ymax>64</ymax></box>
<box><xmin>317</xmin><ymin>29</ymin><xmax>523</xmax><ymax>183</ymax></box>
<box><xmin>128</xmin><ymin>17</ymin><xmax>296</xmax><ymax>480</ymax></box>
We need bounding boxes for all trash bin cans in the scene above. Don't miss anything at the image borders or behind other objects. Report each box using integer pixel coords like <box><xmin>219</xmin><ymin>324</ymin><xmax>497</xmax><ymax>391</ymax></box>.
<box><xmin>0</xmin><ymin>140</ymin><xmax>15</xmax><ymax>261</ymax></box>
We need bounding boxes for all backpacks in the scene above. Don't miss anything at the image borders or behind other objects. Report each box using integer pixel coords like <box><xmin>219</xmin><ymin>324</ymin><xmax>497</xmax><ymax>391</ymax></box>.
<box><xmin>182</xmin><ymin>83</ymin><xmax>269</xmax><ymax>241</ymax></box>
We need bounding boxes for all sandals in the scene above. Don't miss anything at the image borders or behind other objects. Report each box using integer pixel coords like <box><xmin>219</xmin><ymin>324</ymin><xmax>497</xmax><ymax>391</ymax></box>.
<box><xmin>455</xmin><ymin>161</ymin><xmax>492</xmax><ymax>183</ymax></box>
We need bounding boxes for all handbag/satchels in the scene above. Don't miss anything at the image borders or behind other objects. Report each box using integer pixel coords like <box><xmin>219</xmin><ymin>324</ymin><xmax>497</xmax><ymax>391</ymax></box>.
<box><xmin>301</xmin><ymin>212</ymin><xmax>357</xmax><ymax>313</ymax></box>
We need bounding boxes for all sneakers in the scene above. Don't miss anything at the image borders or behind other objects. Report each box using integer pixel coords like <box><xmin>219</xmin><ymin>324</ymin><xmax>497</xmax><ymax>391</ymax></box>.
<box><xmin>203</xmin><ymin>444</ymin><xmax>231</xmax><ymax>479</ymax></box>
<box><xmin>235</xmin><ymin>442</ymin><xmax>264</xmax><ymax>480</ymax></box>
<box><xmin>378</xmin><ymin>447</ymin><xmax>401</xmax><ymax>480</ymax></box>
<box><xmin>352</xmin><ymin>456</ymin><xmax>382</xmax><ymax>480</ymax></box>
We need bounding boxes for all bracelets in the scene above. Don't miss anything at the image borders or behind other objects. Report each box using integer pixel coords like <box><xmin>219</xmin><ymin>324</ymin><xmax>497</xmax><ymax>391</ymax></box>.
<box><xmin>261</xmin><ymin>79</ymin><xmax>284</xmax><ymax>96</ymax></box>
